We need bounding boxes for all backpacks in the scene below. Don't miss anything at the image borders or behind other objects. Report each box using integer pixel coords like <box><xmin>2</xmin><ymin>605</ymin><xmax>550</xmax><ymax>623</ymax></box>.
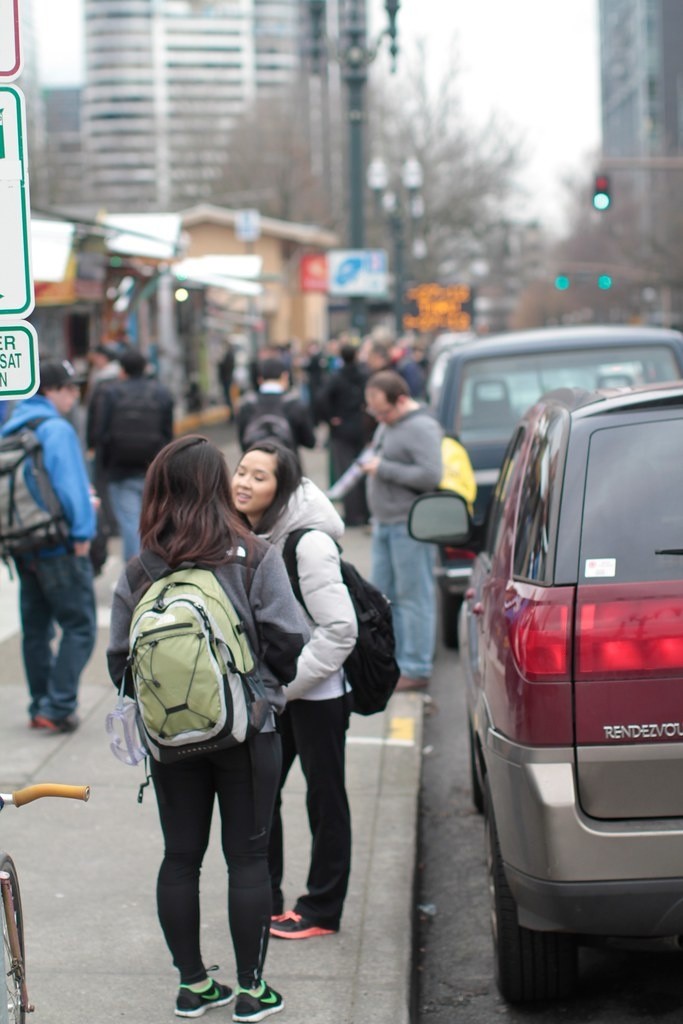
<box><xmin>282</xmin><ymin>528</ymin><xmax>401</xmax><ymax>718</ymax></box>
<box><xmin>0</xmin><ymin>416</ymin><xmax>70</xmax><ymax>560</ymax></box>
<box><xmin>110</xmin><ymin>380</ymin><xmax>162</xmax><ymax>468</ymax></box>
<box><xmin>240</xmin><ymin>391</ymin><xmax>298</xmax><ymax>454</ymax></box>
<box><xmin>120</xmin><ymin>535</ymin><xmax>276</xmax><ymax>797</ymax></box>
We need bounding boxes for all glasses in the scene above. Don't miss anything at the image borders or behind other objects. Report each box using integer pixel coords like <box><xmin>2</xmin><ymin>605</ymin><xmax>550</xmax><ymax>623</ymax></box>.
<box><xmin>106</xmin><ymin>701</ymin><xmax>147</xmax><ymax>766</ymax></box>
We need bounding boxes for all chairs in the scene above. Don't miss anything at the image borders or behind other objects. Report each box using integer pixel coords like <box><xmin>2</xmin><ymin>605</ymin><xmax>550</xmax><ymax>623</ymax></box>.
<box><xmin>596</xmin><ymin>373</ymin><xmax>634</xmax><ymax>391</ymax></box>
<box><xmin>472</xmin><ymin>378</ymin><xmax>510</xmax><ymax>426</ymax></box>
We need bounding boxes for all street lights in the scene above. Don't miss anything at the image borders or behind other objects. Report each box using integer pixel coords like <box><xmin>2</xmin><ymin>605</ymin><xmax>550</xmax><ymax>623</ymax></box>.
<box><xmin>369</xmin><ymin>150</ymin><xmax>426</xmax><ymax>329</ymax></box>
<box><xmin>308</xmin><ymin>0</ymin><xmax>400</xmax><ymax>333</ymax></box>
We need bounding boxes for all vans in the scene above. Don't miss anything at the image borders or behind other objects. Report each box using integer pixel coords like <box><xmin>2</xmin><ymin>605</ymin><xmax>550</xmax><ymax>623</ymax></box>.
<box><xmin>424</xmin><ymin>322</ymin><xmax>683</xmax><ymax>647</ymax></box>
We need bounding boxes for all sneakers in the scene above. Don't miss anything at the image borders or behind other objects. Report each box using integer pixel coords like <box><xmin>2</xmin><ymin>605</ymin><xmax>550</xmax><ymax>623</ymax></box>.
<box><xmin>270</xmin><ymin>911</ymin><xmax>336</xmax><ymax>939</ymax></box>
<box><xmin>233</xmin><ymin>981</ymin><xmax>285</xmax><ymax>1022</ymax></box>
<box><xmin>174</xmin><ymin>977</ymin><xmax>234</xmax><ymax>1017</ymax></box>
<box><xmin>30</xmin><ymin>710</ymin><xmax>83</xmax><ymax>731</ymax></box>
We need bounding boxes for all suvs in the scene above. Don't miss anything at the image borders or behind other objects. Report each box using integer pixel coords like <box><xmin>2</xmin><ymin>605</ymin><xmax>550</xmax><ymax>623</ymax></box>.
<box><xmin>404</xmin><ymin>369</ymin><xmax>683</xmax><ymax>1010</ymax></box>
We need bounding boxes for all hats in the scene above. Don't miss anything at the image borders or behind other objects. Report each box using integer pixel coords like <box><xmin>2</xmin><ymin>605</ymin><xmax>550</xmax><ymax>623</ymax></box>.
<box><xmin>40</xmin><ymin>358</ymin><xmax>87</xmax><ymax>387</ymax></box>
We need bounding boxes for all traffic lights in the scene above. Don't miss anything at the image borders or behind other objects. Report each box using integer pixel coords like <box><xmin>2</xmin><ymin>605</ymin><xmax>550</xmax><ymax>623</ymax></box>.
<box><xmin>593</xmin><ymin>174</ymin><xmax>609</xmax><ymax>211</ymax></box>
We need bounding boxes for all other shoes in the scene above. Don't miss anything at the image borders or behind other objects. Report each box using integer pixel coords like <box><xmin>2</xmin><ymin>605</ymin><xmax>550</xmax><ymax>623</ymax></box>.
<box><xmin>393</xmin><ymin>676</ymin><xmax>428</xmax><ymax>692</ymax></box>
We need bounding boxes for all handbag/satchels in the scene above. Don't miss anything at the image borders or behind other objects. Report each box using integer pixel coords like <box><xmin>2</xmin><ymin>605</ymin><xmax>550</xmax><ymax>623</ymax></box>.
<box><xmin>440</xmin><ymin>436</ymin><xmax>478</xmax><ymax>517</ymax></box>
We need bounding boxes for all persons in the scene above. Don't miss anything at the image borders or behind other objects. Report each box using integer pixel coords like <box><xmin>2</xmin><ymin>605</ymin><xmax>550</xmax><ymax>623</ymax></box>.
<box><xmin>322</xmin><ymin>371</ymin><xmax>445</xmax><ymax>691</ymax></box>
<box><xmin>106</xmin><ymin>435</ymin><xmax>309</xmax><ymax>1024</ymax></box>
<box><xmin>55</xmin><ymin>325</ymin><xmax>428</xmax><ymax>569</ymax></box>
<box><xmin>0</xmin><ymin>359</ymin><xmax>97</xmax><ymax>734</ymax></box>
<box><xmin>228</xmin><ymin>439</ymin><xmax>358</xmax><ymax>939</ymax></box>
